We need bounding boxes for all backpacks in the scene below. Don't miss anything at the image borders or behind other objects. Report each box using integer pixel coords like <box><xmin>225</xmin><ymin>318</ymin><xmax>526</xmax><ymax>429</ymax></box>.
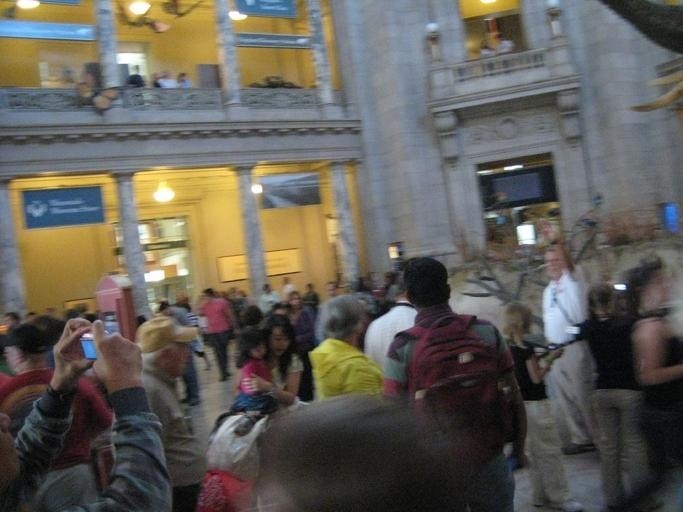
<box><xmin>394</xmin><ymin>314</ymin><xmax>520</xmax><ymax>468</ymax></box>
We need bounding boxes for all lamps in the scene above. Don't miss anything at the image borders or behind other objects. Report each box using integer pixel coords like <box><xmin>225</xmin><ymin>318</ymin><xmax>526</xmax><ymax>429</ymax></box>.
<box><xmin>424</xmin><ymin>21</ymin><xmax>441</xmax><ymax>61</ymax></box>
<box><xmin>543</xmin><ymin>0</ymin><xmax>564</xmax><ymax>40</ymax></box>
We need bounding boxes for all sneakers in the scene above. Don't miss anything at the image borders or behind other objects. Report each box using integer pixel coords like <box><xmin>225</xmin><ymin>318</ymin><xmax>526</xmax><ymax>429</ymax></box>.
<box><xmin>561</xmin><ymin>442</ymin><xmax>596</xmax><ymax>455</ymax></box>
<box><xmin>548</xmin><ymin>498</ymin><xmax>585</xmax><ymax>512</ymax></box>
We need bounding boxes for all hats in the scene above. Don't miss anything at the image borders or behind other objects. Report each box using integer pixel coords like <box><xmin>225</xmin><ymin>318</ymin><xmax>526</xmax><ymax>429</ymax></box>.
<box><xmin>403</xmin><ymin>257</ymin><xmax>450</xmax><ymax>308</ymax></box>
<box><xmin>135</xmin><ymin>315</ymin><xmax>198</xmax><ymax>354</ymax></box>
<box><xmin>1</xmin><ymin>324</ymin><xmax>50</xmax><ymax>355</ymax></box>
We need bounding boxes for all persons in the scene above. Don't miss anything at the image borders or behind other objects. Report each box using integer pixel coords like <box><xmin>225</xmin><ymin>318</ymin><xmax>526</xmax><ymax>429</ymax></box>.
<box><xmin>498</xmin><ymin>296</ymin><xmax>585</xmax><ymax>511</ymax></box>
<box><xmin>576</xmin><ymin>282</ymin><xmax>660</xmax><ymax>511</ymax></box>
<box><xmin>151</xmin><ymin>70</ymin><xmax>169</xmax><ymax>88</ymax></box>
<box><xmin>176</xmin><ymin>72</ymin><xmax>193</xmax><ymax>89</ymax></box>
<box><xmin>383</xmin><ymin>252</ymin><xmax>528</xmax><ymax>508</ymax></box>
<box><xmin>627</xmin><ymin>260</ymin><xmax>682</xmax><ymax>471</ymax></box>
<box><xmin>535</xmin><ymin>243</ymin><xmax>598</xmax><ymax>456</ymax></box>
<box><xmin>126</xmin><ymin>65</ymin><xmax>144</xmax><ymax>106</ymax></box>
<box><xmin>1</xmin><ymin>272</ymin><xmax>529</xmax><ymax>511</ymax></box>
<box><xmin>493</xmin><ymin>32</ymin><xmax>517</xmax><ymax>73</ymax></box>
<box><xmin>478</xmin><ymin>39</ymin><xmax>495</xmax><ymax>73</ymax></box>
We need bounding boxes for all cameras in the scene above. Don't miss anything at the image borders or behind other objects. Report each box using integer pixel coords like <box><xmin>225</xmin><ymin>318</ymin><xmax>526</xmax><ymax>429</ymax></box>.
<box><xmin>613</xmin><ymin>282</ymin><xmax>629</xmax><ymax>292</ymax></box>
<box><xmin>79</xmin><ymin>330</ymin><xmax>110</xmax><ymax>360</ymax></box>
